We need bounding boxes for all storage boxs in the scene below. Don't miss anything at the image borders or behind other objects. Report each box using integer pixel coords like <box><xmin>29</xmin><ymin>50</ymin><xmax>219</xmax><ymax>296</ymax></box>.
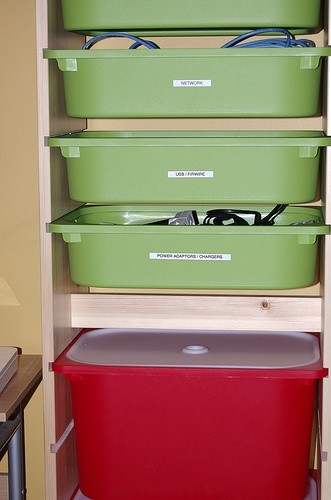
<box><xmin>44</xmin><ymin>47</ymin><xmax>328</xmax><ymax>117</ymax></box>
<box><xmin>61</xmin><ymin>0</ymin><xmax>321</xmax><ymax>35</ymax></box>
<box><xmin>48</xmin><ymin>130</ymin><xmax>331</xmax><ymax>204</ymax></box>
<box><xmin>49</xmin><ymin>329</ymin><xmax>329</xmax><ymax>500</ymax></box>
<box><xmin>50</xmin><ymin>205</ymin><xmax>331</xmax><ymax>290</ymax></box>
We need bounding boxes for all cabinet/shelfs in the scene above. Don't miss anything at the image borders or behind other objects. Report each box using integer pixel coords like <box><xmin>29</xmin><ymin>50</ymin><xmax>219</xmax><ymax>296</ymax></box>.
<box><xmin>34</xmin><ymin>0</ymin><xmax>331</xmax><ymax>500</ymax></box>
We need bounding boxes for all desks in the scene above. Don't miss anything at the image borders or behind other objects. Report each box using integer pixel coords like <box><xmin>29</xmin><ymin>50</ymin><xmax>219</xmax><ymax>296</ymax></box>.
<box><xmin>0</xmin><ymin>354</ymin><xmax>43</xmax><ymax>500</ymax></box>
